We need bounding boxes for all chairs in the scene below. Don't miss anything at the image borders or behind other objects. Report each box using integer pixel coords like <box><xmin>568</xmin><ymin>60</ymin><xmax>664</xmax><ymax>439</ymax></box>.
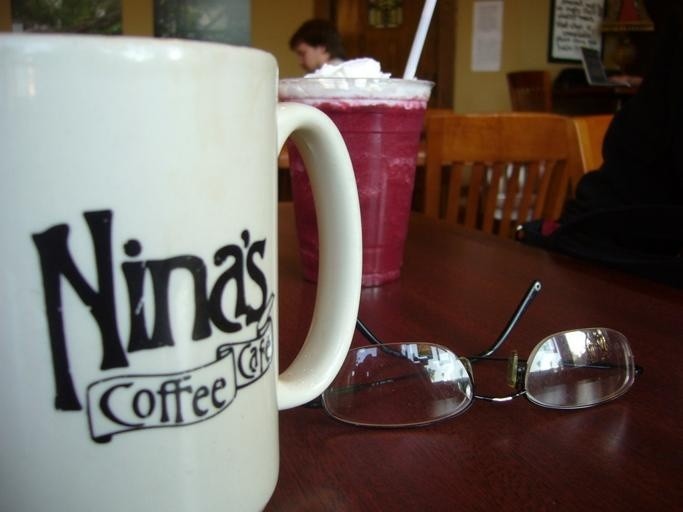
<box><xmin>504</xmin><ymin>69</ymin><xmax>555</xmax><ymax>112</ymax></box>
<box><xmin>423</xmin><ymin>112</ymin><xmax>576</xmax><ymax>242</ymax></box>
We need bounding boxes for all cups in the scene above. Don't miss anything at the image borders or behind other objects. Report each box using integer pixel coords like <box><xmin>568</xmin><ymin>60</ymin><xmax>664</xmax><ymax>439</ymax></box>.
<box><xmin>2</xmin><ymin>34</ymin><xmax>362</xmax><ymax>512</ymax></box>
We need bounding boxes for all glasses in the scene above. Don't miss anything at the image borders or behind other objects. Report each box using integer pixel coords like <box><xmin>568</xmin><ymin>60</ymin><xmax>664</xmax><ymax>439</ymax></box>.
<box><xmin>306</xmin><ymin>279</ymin><xmax>639</xmax><ymax>430</ymax></box>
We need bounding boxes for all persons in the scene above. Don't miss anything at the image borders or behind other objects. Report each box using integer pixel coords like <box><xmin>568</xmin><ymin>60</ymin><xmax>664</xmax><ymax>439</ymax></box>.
<box><xmin>514</xmin><ymin>0</ymin><xmax>683</xmax><ymax>291</ymax></box>
<box><xmin>290</xmin><ymin>18</ymin><xmax>346</xmax><ymax>73</ymax></box>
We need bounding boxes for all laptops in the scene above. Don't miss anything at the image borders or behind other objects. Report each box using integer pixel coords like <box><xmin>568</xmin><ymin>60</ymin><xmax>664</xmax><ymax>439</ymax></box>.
<box><xmin>579</xmin><ymin>46</ymin><xmax>630</xmax><ymax>88</ymax></box>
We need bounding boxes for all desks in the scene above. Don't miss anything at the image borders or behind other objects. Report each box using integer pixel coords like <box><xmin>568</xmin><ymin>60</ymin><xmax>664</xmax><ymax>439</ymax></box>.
<box><xmin>227</xmin><ymin>199</ymin><xmax>682</xmax><ymax>511</ymax></box>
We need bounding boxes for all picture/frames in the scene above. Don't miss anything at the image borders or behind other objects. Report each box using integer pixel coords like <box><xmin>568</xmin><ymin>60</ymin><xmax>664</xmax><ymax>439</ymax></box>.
<box><xmin>578</xmin><ymin>45</ymin><xmax>609</xmax><ymax>85</ymax></box>
<box><xmin>547</xmin><ymin>0</ymin><xmax>609</xmax><ymax>65</ymax></box>
